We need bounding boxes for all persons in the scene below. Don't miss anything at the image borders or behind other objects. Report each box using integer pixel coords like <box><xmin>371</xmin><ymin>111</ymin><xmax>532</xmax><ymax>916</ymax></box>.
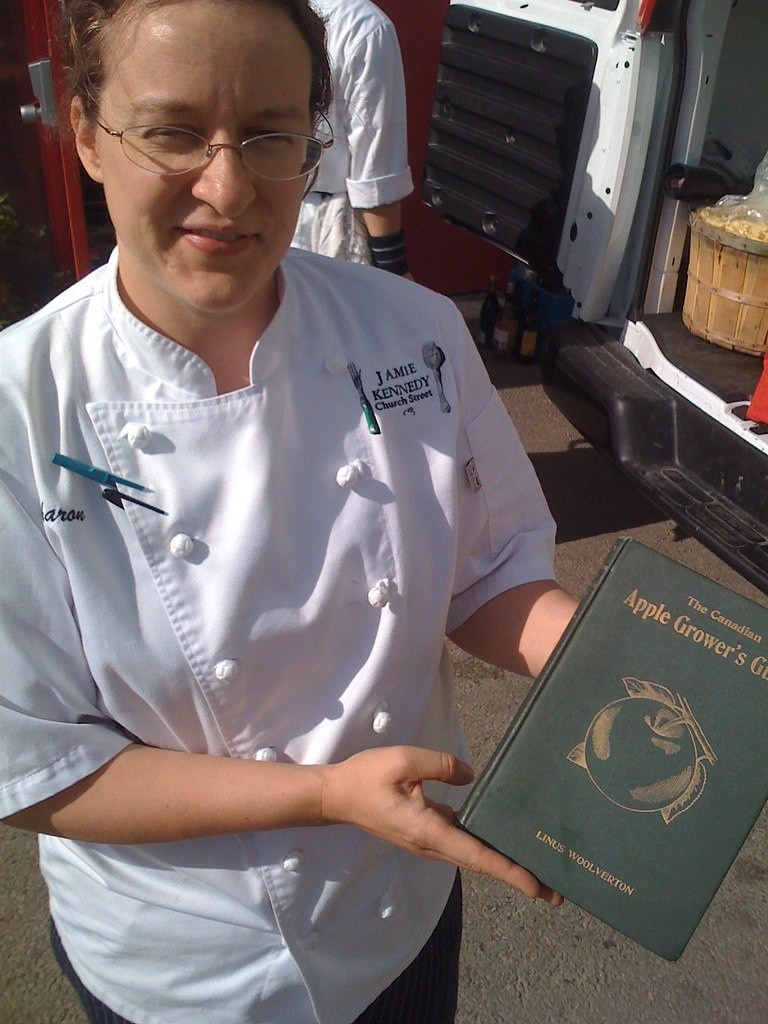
<box><xmin>289</xmin><ymin>0</ymin><xmax>414</xmax><ymax>281</ymax></box>
<box><xmin>0</xmin><ymin>0</ymin><xmax>580</xmax><ymax>1024</ymax></box>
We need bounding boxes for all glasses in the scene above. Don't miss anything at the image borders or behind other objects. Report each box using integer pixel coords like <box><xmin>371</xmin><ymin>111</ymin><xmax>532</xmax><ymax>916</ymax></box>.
<box><xmin>93</xmin><ymin>101</ymin><xmax>334</xmax><ymax>181</ymax></box>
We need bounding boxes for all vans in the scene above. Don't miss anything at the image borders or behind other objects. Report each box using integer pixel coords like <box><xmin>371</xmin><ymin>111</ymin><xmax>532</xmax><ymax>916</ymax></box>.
<box><xmin>417</xmin><ymin>1</ymin><xmax>768</xmax><ymax>585</ymax></box>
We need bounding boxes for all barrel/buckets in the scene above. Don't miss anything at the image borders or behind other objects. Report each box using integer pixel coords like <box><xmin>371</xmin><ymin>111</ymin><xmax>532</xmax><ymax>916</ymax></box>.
<box><xmin>681</xmin><ymin>214</ymin><xmax>767</xmax><ymax>357</ymax></box>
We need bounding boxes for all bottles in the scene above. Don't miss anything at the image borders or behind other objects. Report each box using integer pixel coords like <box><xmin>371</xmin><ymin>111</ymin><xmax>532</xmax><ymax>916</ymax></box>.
<box><xmin>479</xmin><ymin>276</ymin><xmax>500</xmax><ymax>336</ymax></box>
<box><xmin>515</xmin><ymin>289</ymin><xmax>544</xmax><ymax>362</ymax></box>
<box><xmin>489</xmin><ymin>280</ymin><xmax>521</xmax><ymax>354</ymax></box>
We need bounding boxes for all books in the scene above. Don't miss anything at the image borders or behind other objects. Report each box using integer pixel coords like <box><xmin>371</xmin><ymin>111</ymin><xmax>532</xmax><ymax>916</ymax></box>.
<box><xmin>456</xmin><ymin>536</ymin><xmax>768</xmax><ymax>962</ymax></box>
